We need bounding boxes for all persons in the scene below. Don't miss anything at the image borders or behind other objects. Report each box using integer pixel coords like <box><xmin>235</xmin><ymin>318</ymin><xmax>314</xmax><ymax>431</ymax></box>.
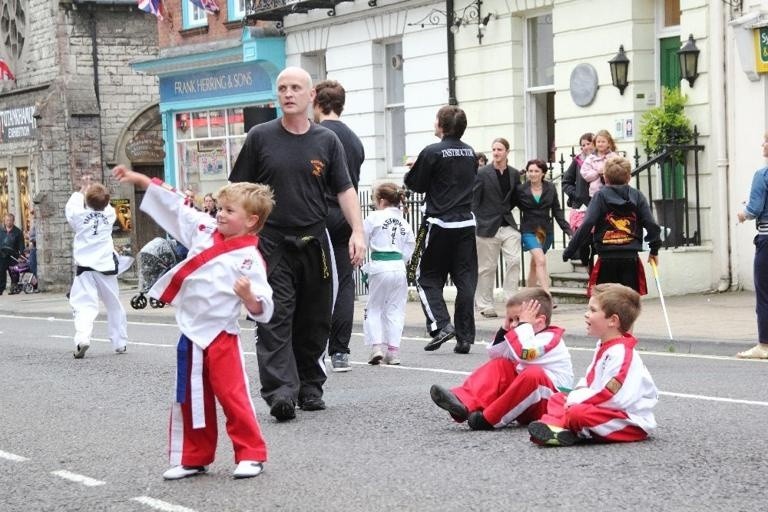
<box><xmin>65</xmin><ymin>174</ymin><xmax>127</xmax><ymax>358</ymax></box>
<box><xmin>0</xmin><ymin>213</ymin><xmax>25</xmax><ymax>295</ymax></box>
<box><xmin>737</xmin><ymin>131</ymin><xmax>768</xmax><ymax>358</ymax></box>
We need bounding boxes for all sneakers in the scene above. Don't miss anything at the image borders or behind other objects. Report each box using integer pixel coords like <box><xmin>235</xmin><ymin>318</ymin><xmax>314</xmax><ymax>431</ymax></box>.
<box><xmin>297</xmin><ymin>394</ymin><xmax>325</xmax><ymax>410</ymax></box>
<box><xmin>430</xmin><ymin>384</ymin><xmax>466</xmax><ymax>421</ymax></box>
<box><xmin>73</xmin><ymin>343</ymin><xmax>89</xmax><ymax>358</ymax></box>
<box><xmin>425</xmin><ymin>324</ymin><xmax>456</xmax><ymax>351</ymax></box>
<box><xmin>737</xmin><ymin>346</ymin><xmax>768</xmax><ymax>359</ymax></box>
<box><xmin>529</xmin><ymin>421</ymin><xmax>575</xmax><ymax>446</ymax></box>
<box><xmin>453</xmin><ymin>340</ymin><xmax>469</xmax><ymax>353</ymax></box>
<box><xmin>369</xmin><ymin>348</ymin><xmax>401</xmax><ymax>364</ymax></box>
<box><xmin>271</xmin><ymin>397</ymin><xmax>296</xmax><ymax>422</ymax></box>
<box><xmin>468</xmin><ymin>411</ymin><xmax>493</xmax><ymax>430</ymax></box>
<box><xmin>233</xmin><ymin>460</ymin><xmax>264</xmax><ymax>478</ymax></box>
<box><xmin>330</xmin><ymin>353</ymin><xmax>351</xmax><ymax>372</ymax></box>
<box><xmin>163</xmin><ymin>465</ymin><xmax>209</xmax><ymax>480</ymax></box>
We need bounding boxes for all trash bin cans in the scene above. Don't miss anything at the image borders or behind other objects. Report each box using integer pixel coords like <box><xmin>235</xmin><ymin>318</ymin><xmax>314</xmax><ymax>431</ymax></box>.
<box><xmin>652</xmin><ymin>197</ymin><xmax>685</xmax><ymax>247</ymax></box>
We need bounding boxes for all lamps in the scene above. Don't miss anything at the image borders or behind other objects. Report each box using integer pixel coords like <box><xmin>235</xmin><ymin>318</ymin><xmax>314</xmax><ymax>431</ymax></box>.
<box><xmin>608</xmin><ymin>44</ymin><xmax>630</xmax><ymax>94</ymax></box>
<box><xmin>477</xmin><ymin>11</ymin><xmax>499</xmax><ymax>34</ymax></box>
<box><xmin>449</xmin><ymin>0</ymin><xmax>480</xmax><ymax>35</ymax></box>
<box><xmin>678</xmin><ymin>34</ymin><xmax>702</xmax><ymax>88</ymax></box>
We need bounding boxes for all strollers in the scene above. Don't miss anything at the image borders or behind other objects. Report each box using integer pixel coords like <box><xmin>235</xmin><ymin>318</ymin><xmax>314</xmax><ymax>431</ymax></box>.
<box><xmin>130</xmin><ymin>236</ymin><xmax>188</xmax><ymax>310</ymax></box>
<box><xmin>9</xmin><ymin>247</ymin><xmax>37</xmax><ymax>295</ymax></box>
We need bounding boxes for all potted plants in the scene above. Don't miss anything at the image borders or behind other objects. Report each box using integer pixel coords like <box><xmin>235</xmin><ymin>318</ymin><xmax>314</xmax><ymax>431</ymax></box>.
<box><xmin>638</xmin><ymin>87</ymin><xmax>699</xmax><ymax>245</ymax></box>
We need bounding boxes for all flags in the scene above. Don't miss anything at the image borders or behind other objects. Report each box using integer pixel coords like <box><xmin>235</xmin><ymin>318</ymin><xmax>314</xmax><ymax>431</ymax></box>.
<box><xmin>0</xmin><ymin>52</ymin><xmax>16</xmax><ymax>81</ymax></box>
<box><xmin>137</xmin><ymin>0</ymin><xmax>165</xmax><ymax>21</ymax></box>
<box><xmin>190</xmin><ymin>0</ymin><xmax>219</xmax><ymax>14</ymax></box>
